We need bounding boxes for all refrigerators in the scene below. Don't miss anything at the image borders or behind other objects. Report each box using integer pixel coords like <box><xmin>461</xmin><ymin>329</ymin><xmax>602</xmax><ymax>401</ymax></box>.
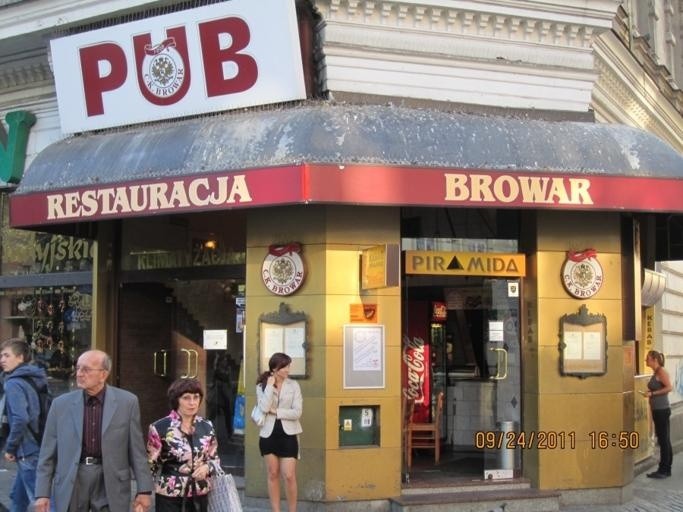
<box><xmin>401</xmin><ymin>298</ymin><xmax>449</xmax><ymax>452</ymax></box>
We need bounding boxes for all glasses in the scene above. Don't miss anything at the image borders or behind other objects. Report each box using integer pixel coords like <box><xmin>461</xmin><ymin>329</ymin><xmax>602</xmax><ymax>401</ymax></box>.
<box><xmin>76</xmin><ymin>367</ymin><xmax>106</xmax><ymax>374</ymax></box>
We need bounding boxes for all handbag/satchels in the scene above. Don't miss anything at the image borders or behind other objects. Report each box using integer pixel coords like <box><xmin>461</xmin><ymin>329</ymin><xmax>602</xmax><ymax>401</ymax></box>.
<box><xmin>206</xmin><ymin>460</ymin><xmax>244</xmax><ymax>512</ymax></box>
<box><xmin>251</xmin><ymin>405</ymin><xmax>266</xmax><ymax>428</ymax></box>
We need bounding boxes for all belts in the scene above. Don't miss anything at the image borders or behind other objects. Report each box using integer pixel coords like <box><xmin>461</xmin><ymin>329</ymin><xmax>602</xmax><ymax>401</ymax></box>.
<box><xmin>81</xmin><ymin>457</ymin><xmax>102</xmax><ymax>466</ymax></box>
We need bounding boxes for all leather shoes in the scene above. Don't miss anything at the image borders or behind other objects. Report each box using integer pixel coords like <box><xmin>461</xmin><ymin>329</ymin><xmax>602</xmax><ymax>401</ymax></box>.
<box><xmin>646</xmin><ymin>473</ymin><xmax>665</xmax><ymax>479</ymax></box>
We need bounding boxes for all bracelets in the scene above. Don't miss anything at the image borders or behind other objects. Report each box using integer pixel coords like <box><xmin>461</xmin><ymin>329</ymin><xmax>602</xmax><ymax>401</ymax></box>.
<box><xmin>651</xmin><ymin>391</ymin><xmax>653</xmax><ymax>396</ymax></box>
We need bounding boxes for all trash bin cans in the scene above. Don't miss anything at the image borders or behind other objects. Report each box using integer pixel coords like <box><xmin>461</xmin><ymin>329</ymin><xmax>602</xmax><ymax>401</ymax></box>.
<box><xmin>496</xmin><ymin>419</ymin><xmax>520</xmax><ymax>477</ymax></box>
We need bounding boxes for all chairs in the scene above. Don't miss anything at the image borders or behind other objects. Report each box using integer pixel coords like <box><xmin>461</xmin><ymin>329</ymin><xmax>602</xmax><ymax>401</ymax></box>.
<box><xmin>407</xmin><ymin>392</ymin><xmax>444</xmax><ymax>466</ymax></box>
<box><xmin>401</xmin><ymin>397</ymin><xmax>416</xmax><ymax>468</ymax></box>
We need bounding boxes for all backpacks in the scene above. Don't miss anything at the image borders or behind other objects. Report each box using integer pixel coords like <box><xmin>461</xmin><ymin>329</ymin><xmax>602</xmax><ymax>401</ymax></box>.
<box><xmin>22</xmin><ymin>376</ymin><xmax>55</xmax><ymax>448</ymax></box>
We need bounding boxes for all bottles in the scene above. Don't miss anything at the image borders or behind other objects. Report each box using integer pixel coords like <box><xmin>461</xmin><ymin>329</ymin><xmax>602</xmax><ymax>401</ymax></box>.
<box><xmin>432</xmin><ymin>395</ymin><xmax>437</xmax><ymax>422</ymax></box>
<box><xmin>432</xmin><ymin>327</ymin><xmax>438</xmax><ymax>344</ymax></box>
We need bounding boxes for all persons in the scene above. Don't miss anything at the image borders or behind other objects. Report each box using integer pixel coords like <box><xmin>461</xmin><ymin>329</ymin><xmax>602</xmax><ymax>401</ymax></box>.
<box><xmin>642</xmin><ymin>351</ymin><xmax>673</xmax><ymax>479</ymax></box>
<box><xmin>256</xmin><ymin>353</ymin><xmax>303</xmax><ymax>512</ymax></box>
<box><xmin>214</xmin><ymin>355</ymin><xmax>238</xmax><ymax>439</ymax></box>
<box><xmin>132</xmin><ymin>378</ymin><xmax>221</xmax><ymax>512</ymax></box>
<box><xmin>0</xmin><ymin>338</ymin><xmax>56</xmax><ymax>512</ymax></box>
<box><xmin>35</xmin><ymin>350</ymin><xmax>153</xmax><ymax>512</ymax></box>
